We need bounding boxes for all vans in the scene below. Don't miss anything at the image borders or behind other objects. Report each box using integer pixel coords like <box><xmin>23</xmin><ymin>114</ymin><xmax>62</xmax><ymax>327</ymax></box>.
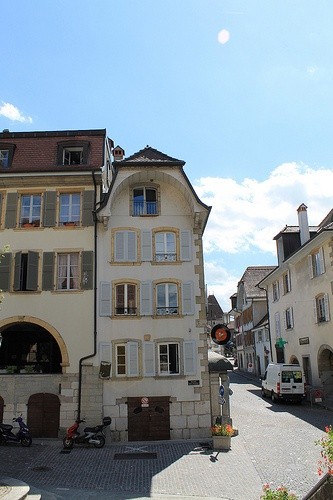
<box><xmin>258</xmin><ymin>362</ymin><xmax>304</xmax><ymax>403</ymax></box>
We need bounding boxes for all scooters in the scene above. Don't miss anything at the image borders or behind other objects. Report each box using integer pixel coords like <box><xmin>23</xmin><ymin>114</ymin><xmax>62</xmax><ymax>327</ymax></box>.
<box><xmin>63</xmin><ymin>416</ymin><xmax>111</xmax><ymax>448</ymax></box>
<box><xmin>0</xmin><ymin>413</ymin><xmax>33</xmax><ymax>448</ymax></box>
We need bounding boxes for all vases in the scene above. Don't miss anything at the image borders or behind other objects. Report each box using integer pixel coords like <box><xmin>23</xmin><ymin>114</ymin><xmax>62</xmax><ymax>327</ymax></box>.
<box><xmin>24</xmin><ymin>224</ymin><xmax>32</xmax><ymax>228</ymax></box>
<box><xmin>66</xmin><ymin>223</ymin><xmax>74</xmax><ymax>229</ymax></box>
<box><xmin>213</xmin><ymin>436</ymin><xmax>230</xmax><ymax>449</ymax></box>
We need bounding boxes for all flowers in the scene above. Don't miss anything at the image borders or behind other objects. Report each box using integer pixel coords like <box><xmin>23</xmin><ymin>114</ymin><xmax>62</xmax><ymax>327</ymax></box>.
<box><xmin>210</xmin><ymin>423</ymin><xmax>234</xmax><ymax>437</ymax></box>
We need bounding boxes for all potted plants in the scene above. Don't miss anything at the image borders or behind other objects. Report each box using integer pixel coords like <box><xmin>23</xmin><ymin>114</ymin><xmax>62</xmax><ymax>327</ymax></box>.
<box><xmin>0</xmin><ymin>366</ymin><xmax>17</xmax><ymax>373</ymax></box>
<box><xmin>20</xmin><ymin>365</ymin><xmax>42</xmax><ymax>373</ymax></box>
